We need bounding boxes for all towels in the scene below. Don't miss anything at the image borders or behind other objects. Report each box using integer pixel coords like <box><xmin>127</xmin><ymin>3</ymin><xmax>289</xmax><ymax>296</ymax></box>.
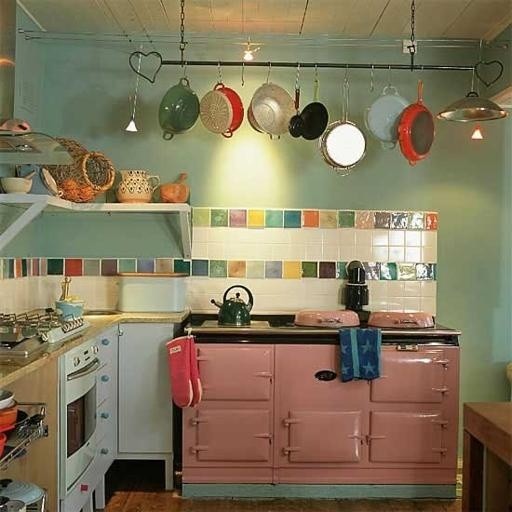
<box><xmin>336</xmin><ymin>327</ymin><xmax>383</xmax><ymax>383</ymax></box>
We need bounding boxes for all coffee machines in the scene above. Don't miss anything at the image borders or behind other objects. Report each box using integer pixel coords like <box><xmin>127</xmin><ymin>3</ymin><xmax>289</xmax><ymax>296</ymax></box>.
<box><xmin>344</xmin><ymin>259</ymin><xmax>371</xmax><ymax>323</ymax></box>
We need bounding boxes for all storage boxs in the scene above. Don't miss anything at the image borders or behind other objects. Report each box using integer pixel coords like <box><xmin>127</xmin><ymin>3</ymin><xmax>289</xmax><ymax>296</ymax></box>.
<box><xmin>116</xmin><ymin>272</ymin><xmax>190</xmax><ymax>314</ymax></box>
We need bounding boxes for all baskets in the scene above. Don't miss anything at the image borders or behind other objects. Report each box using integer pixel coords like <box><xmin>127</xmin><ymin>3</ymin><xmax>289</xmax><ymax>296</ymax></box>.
<box><xmin>38</xmin><ymin>136</ymin><xmax>115</xmax><ymax>203</ymax></box>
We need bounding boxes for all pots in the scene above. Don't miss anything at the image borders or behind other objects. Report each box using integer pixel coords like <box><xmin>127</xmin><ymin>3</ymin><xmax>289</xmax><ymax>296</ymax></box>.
<box><xmin>246</xmin><ymin>82</ymin><xmax>296</xmax><ymax>142</ymax></box>
<box><xmin>158</xmin><ymin>77</ymin><xmax>201</xmax><ymax>141</ymax></box>
<box><xmin>397</xmin><ymin>79</ymin><xmax>435</xmax><ymax>168</ymax></box>
<box><xmin>363</xmin><ymin>84</ymin><xmax>409</xmax><ymax>152</ymax></box>
<box><xmin>201</xmin><ymin>81</ymin><xmax>240</xmax><ymax>139</ymax></box>
<box><xmin>319</xmin><ymin>80</ymin><xmax>368</xmax><ymax>178</ymax></box>
<box><xmin>300</xmin><ymin>77</ymin><xmax>328</xmax><ymax>143</ymax></box>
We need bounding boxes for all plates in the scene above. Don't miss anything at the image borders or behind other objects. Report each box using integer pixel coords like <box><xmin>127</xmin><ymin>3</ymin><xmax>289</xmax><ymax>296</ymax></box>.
<box><xmin>0</xmin><ymin>389</ymin><xmax>15</xmax><ymax>410</ymax></box>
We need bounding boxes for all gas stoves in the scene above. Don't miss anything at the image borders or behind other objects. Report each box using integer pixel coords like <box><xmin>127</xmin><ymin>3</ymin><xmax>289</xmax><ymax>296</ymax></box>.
<box><xmin>0</xmin><ymin>312</ymin><xmax>92</xmax><ymax>345</ymax></box>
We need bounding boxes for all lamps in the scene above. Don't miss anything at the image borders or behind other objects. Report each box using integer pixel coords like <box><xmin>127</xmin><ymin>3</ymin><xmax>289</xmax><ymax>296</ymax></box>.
<box><xmin>472</xmin><ymin>120</ymin><xmax>483</xmax><ymax>141</ymax></box>
<box><xmin>243</xmin><ymin>41</ymin><xmax>259</xmax><ymax>61</ymax></box>
<box><xmin>124</xmin><ymin>41</ymin><xmax>144</xmax><ymax>134</ymax></box>
<box><xmin>435</xmin><ymin>69</ymin><xmax>507</xmax><ymax>121</ymax></box>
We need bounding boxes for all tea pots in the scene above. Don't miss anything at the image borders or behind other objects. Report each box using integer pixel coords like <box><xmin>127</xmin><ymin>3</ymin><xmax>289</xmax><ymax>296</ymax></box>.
<box><xmin>211</xmin><ymin>285</ymin><xmax>254</xmax><ymax>328</ymax></box>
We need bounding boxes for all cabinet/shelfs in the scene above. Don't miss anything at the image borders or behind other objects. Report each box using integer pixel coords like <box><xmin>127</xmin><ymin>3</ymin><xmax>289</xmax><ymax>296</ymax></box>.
<box><xmin>119</xmin><ymin>323</ymin><xmax>175</xmax><ymax>497</ymax></box>
<box><xmin>96</xmin><ymin>325</ymin><xmax>119</xmax><ymax>480</ymax></box>
<box><xmin>460</xmin><ymin>399</ymin><xmax>511</xmax><ymax>511</ymax></box>
<box><xmin>1</xmin><ymin>353</ymin><xmax>61</xmax><ymax>511</ymax></box>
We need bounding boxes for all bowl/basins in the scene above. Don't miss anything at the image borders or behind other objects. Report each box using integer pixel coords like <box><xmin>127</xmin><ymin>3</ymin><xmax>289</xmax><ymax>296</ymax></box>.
<box><xmin>1</xmin><ymin>177</ymin><xmax>34</xmax><ymax>193</ymax></box>
<box><xmin>0</xmin><ymin>433</ymin><xmax>9</xmax><ymax>457</ymax></box>
<box><xmin>1</xmin><ymin>400</ymin><xmax>20</xmax><ymax>426</ymax></box>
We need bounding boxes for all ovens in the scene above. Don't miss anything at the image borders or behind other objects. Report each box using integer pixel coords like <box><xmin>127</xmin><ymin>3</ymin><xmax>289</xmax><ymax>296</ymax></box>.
<box><xmin>56</xmin><ymin>339</ymin><xmax>101</xmax><ymax>499</ymax></box>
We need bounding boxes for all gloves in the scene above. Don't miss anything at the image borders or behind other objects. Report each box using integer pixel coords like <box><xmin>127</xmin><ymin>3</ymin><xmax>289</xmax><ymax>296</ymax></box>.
<box><xmin>165</xmin><ymin>335</ymin><xmax>194</xmax><ymax>409</ymax></box>
<box><xmin>189</xmin><ymin>334</ymin><xmax>202</xmax><ymax>408</ymax></box>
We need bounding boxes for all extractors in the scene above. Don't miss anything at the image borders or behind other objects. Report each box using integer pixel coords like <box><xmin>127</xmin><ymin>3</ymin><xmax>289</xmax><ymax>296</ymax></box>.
<box><xmin>0</xmin><ymin>0</ymin><xmax>75</xmax><ymax>166</ymax></box>
